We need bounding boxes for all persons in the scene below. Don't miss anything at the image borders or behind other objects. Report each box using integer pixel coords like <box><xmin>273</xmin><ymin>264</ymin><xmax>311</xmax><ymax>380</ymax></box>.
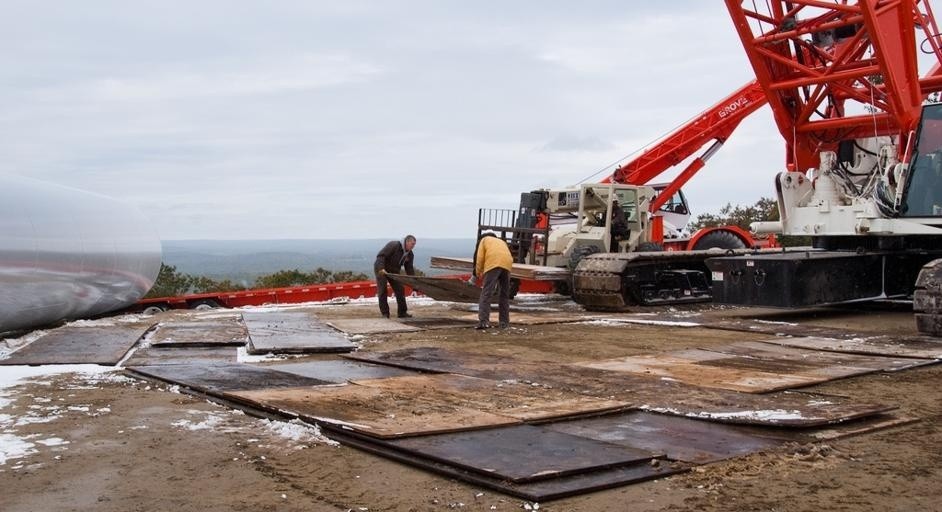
<box><xmin>599</xmin><ymin>192</ymin><xmax>628</xmax><ymax>254</ymax></box>
<box><xmin>467</xmin><ymin>232</ymin><xmax>513</xmax><ymax>331</ymax></box>
<box><xmin>374</xmin><ymin>235</ymin><xmax>421</xmax><ymax>318</ymax></box>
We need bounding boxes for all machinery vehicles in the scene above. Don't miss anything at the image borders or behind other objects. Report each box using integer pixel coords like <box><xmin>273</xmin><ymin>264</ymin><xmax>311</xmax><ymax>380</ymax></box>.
<box><xmin>429</xmin><ymin>56</ymin><xmax>792</xmax><ymax>338</ymax></box>
<box><xmin>704</xmin><ymin>0</ymin><xmax>941</xmax><ymax>339</ymax></box>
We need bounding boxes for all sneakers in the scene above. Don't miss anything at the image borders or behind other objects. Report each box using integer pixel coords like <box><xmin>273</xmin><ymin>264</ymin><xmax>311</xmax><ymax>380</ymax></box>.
<box><xmin>398</xmin><ymin>312</ymin><xmax>412</xmax><ymax>317</ymax></box>
<box><xmin>383</xmin><ymin>312</ymin><xmax>390</xmax><ymax>317</ymax></box>
<box><xmin>474</xmin><ymin>320</ymin><xmax>491</xmax><ymax>329</ymax></box>
<box><xmin>499</xmin><ymin>321</ymin><xmax>507</xmax><ymax>328</ymax></box>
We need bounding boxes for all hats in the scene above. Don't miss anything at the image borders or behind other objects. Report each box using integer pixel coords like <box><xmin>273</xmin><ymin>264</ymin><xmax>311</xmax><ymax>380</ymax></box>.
<box><xmin>481</xmin><ymin>229</ymin><xmax>496</xmax><ymax>236</ymax></box>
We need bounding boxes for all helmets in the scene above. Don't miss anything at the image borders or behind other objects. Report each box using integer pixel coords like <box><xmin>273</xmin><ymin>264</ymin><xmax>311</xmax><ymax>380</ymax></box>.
<box><xmin>607</xmin><ymin>194</ymin><xmax>619</xmax><ymax>202</ymax></box>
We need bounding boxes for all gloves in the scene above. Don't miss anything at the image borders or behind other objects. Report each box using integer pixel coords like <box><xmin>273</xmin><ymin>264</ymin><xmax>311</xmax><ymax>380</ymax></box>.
<box><xmin>467</xmin><ymin>276</ymin><xmax>476</xmax><ymax>286</ymax></box>
<box><xmin>377</xmin><ymin>268</ymin><xmax>388</xmax><ymax>279</ymax></box>
